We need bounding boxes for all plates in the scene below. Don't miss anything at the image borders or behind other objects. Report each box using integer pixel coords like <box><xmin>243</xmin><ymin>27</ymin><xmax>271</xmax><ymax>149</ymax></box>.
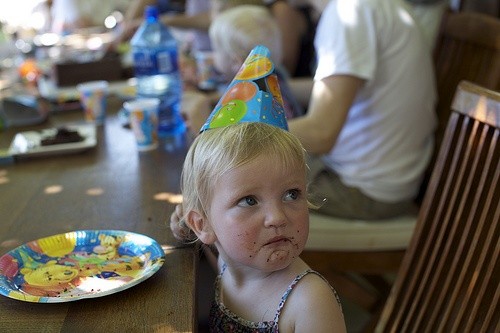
<box><xmin>0</xmin><ymin>229</ymin><xmax>166</xmax><ymax>303</ymax></box>
<box><xmin>11</xmin><ymin>125</ymin><xmax>97</xmax><ymax>157</ymax></box>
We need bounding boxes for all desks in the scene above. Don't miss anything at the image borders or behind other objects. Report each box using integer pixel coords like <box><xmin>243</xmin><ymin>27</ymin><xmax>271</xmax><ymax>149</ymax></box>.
<box><xmin>0</xmin><ymin>24</ymin><xmax>209</xmax><ymax>333</ymax></box>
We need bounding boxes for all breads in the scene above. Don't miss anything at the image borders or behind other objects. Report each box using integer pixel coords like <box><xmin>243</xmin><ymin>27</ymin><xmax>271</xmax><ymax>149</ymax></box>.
<box><xmin>40</xmin><ymin>127</ymin><xmax>83</xmax><ymax>145</ymax></box>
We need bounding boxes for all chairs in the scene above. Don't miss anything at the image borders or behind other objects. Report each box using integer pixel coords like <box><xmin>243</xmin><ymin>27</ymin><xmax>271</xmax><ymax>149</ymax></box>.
<box><xmin>373</xmin><ymin>81</ymin><xmax>500</xmax><ymax>333</ymax></box>
<box><xmin>302</xmin><ymin>9</ymin><xmax>500</xmax><ymax>333</ymax></box>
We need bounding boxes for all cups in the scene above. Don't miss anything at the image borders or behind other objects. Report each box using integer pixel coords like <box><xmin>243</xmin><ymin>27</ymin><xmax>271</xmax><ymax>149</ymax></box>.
<box><xmin>77</xmin><ymin>80</ymin><xmax>108</xmax><ymax>127</ymax></box>
<box><xmin>194</xmin><ymin>51</ymin><xmax>218</xmax><ymax>91</ymax></box>
<box><xmin>122</xmin><ymin>97</ymin><xmax>160</xmax><ymax>153</ymax></box>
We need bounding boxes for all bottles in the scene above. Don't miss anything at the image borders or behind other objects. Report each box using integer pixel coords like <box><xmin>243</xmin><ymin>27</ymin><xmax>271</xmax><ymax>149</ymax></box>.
<box><xmin>132</xmin><ymin>7</ymin><xmax>183</xmax><ymax>153</ymax></box>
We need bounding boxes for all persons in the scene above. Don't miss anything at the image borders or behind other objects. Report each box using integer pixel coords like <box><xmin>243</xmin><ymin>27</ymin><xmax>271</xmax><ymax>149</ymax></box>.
<box><xmin>170</xmin><ymin>46</ymin><xmax>347</xmax><ymax>333</ymax></box>
<box><xmin>114</xmin><ymin>0</ymin><xmax>440</xmax><ymax>277</ymax></box>
